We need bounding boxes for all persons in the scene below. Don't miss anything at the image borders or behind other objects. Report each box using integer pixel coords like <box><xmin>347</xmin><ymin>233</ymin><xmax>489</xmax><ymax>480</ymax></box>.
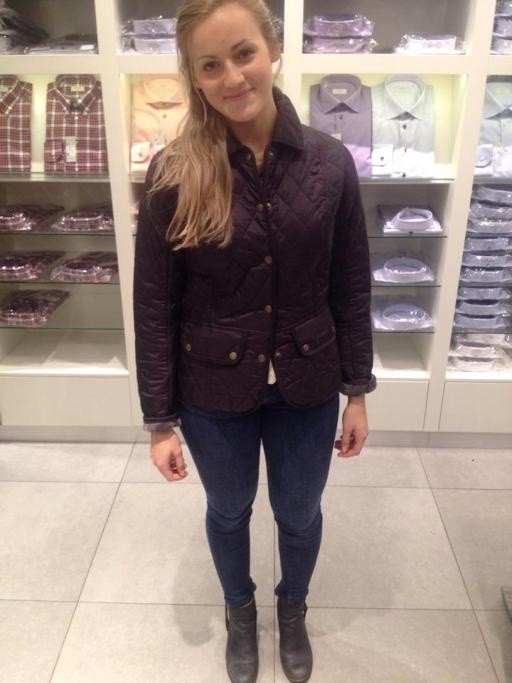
<box><xmin>132</xmin><ymin>0</ymin><xmax>381</xmax><ymax>683</ymax></box>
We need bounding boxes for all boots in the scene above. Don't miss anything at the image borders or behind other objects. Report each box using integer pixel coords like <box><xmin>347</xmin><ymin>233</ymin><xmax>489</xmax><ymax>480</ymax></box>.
<box><xmin>277</xmin><ymin>594</ymin><xmax>312</xmax><ymax>682</ymax></box>
<box><xmin>225</xmin><ymin>596</ymin><xmax>258</xmax><ymax>682</ymax></box>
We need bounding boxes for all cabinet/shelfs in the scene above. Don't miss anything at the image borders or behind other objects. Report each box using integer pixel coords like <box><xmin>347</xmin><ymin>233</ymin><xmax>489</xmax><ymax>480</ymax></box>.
<box><xmin>0</xmin><ymin>0</ymin><xmax>512</xmax><ymax>449</ymax></box>
